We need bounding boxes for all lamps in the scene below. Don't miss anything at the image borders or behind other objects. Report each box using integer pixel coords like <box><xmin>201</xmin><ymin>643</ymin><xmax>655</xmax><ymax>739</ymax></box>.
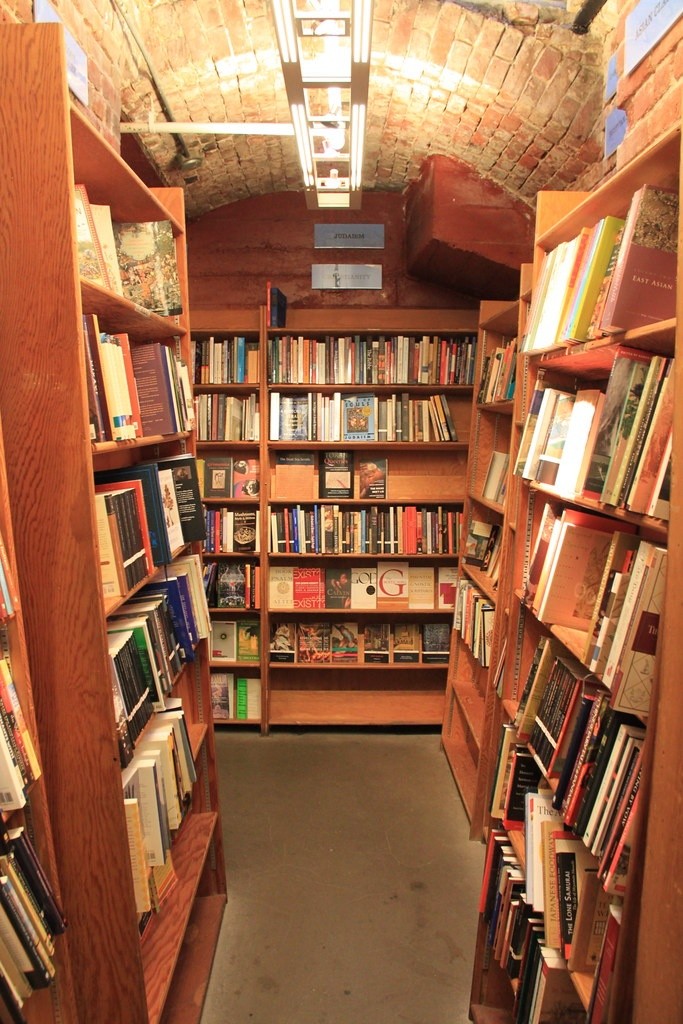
<box><xmin>270</xmin><ymin>0</ymin><xmax>375</xmax><ymax>211</ymax></box>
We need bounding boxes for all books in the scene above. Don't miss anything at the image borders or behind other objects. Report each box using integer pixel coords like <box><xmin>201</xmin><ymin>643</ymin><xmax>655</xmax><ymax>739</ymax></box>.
<box><xmin>453</xmin><ymin>184</ymin><xmax>681</xmax><ymax>1024</ymax></box>
<box><xmin>191</xmin><ymin>286</ymin><xmax>477</xmax><ymax>722</ymax></box>
<box><xmin>64</xmin><ymin>180</ymin><xmax>216</xmax><ymax>941</ymax></box>
<box><xmin>0</xmin><ymin>519</ymin><xmax>66</xmax><ymax>1024</ymax></box>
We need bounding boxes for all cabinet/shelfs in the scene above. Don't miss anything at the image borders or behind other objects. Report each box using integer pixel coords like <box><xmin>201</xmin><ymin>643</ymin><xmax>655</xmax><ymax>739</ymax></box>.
<box><xmin>0</xmin><ymin>427</ymin><xmax>79</xmax><ymax>1024</ymax></box>
<box><xmin>468</xmin><ymin>119</ymin><xmax>683</xmax><ymax>1024</ymax></box>
<box><xmin>1</xmin><ymin>23</ymin><xmax>229</xmax><ymax>1024</ymax></box>
<box><xmin>439</xmin><ymin>265</ymin><xmax>536</xmax><ymax>844</ymax></box>
<box><xmin>190</xmin><ymin>282</ymin><xmax>481</xmax><ymax>738</ymax></box>
